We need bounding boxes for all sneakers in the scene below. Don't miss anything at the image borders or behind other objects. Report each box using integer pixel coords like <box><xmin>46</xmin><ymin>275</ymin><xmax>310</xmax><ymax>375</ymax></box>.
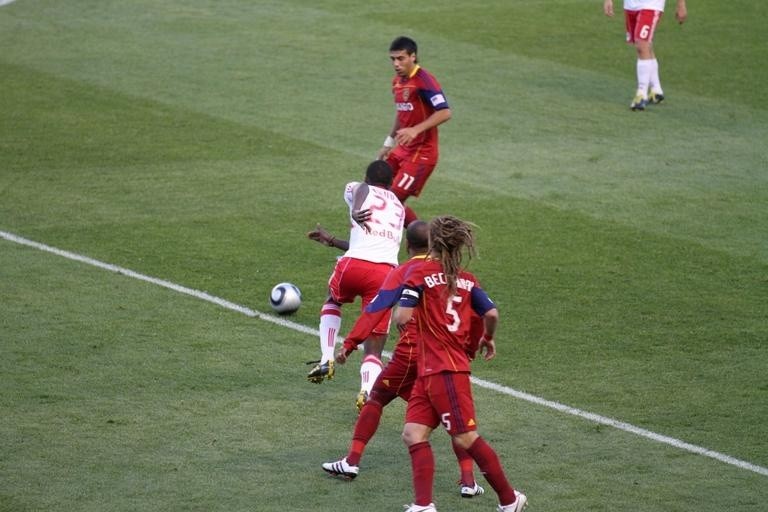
<box><xmin>404</xmin><ymin>503</ymin><xmax>437</xmax><ymax>512</ymax></box>
<box><xmin>461</xmin><ymin>480</ymin><xmax>485</xmax><ymax>497</ymax></box>
<box><xmin>355</xmin><ymin>390</ymin><xmax>368</xmax><ymax>417</ymax></box>
<box><xmin>650</xmin><ymin>92</ymin><xmax>666</xmax><ymax>105</ymax></box>
<box><xmin>306</xmin><ymin>360</ymin><xmax>336</xmax><ymax>383</ymax></box>
<box><xmin>496</xmin><ymin>489</ymin><xmax>529</xmax><ymax>512</ymax></box>
<box><xmin>321</xmin><ymin>456</ymin><xmax>360</xmax><ymax>480</ymax></box>
<box><xmin>630</xmin><ymin>95</ymin><xmax>650</xmax><ymax>111</ymax></box>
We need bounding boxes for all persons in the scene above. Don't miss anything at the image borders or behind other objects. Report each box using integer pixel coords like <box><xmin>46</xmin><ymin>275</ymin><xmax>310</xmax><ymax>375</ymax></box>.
<box><xmin>303</xmin><ymin>157</ymin><xmax>410</xmax><ymax>416</ymax></box>
<box><xmin>374</xmin><ymin>34</ymin><xmax>451</xmax><ymax>231</ymax></box>
<box><xmin>321</xmin><ymin>217</ymin><xmax>490</xmax><ymax>499</ymax></box>
<box><xmin>391</xmin><ymin>214</ymin><xmax>531</xmax><ymax>511</ymax></box>
<box><xmin>604</xmin><ymin>0</ymin><xmax>691</xmax><ymax>110</ymax></box>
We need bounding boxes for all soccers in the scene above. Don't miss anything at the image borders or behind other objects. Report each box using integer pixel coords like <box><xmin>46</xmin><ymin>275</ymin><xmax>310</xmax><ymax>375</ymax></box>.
<box><xmin>269</xmin><ymin>282</ymin><xmax>302</xmax><ymax>316</ymax></box>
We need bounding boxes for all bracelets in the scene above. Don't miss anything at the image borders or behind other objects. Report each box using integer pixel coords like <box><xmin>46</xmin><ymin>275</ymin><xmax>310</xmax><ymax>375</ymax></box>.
<box><xmin>383</xmin><ymin>136</ymin><xmax>397</xmax><ymax>148</ymax></box>
<box><xmin>328</xmin><ymin>236</ymin><xmax>337</xmax><ymax>247</ymax></box>
<box><xmin>482</xmin><ymin>331</ymin><xmax>494</xmax><ymax>343</ymax></box>
